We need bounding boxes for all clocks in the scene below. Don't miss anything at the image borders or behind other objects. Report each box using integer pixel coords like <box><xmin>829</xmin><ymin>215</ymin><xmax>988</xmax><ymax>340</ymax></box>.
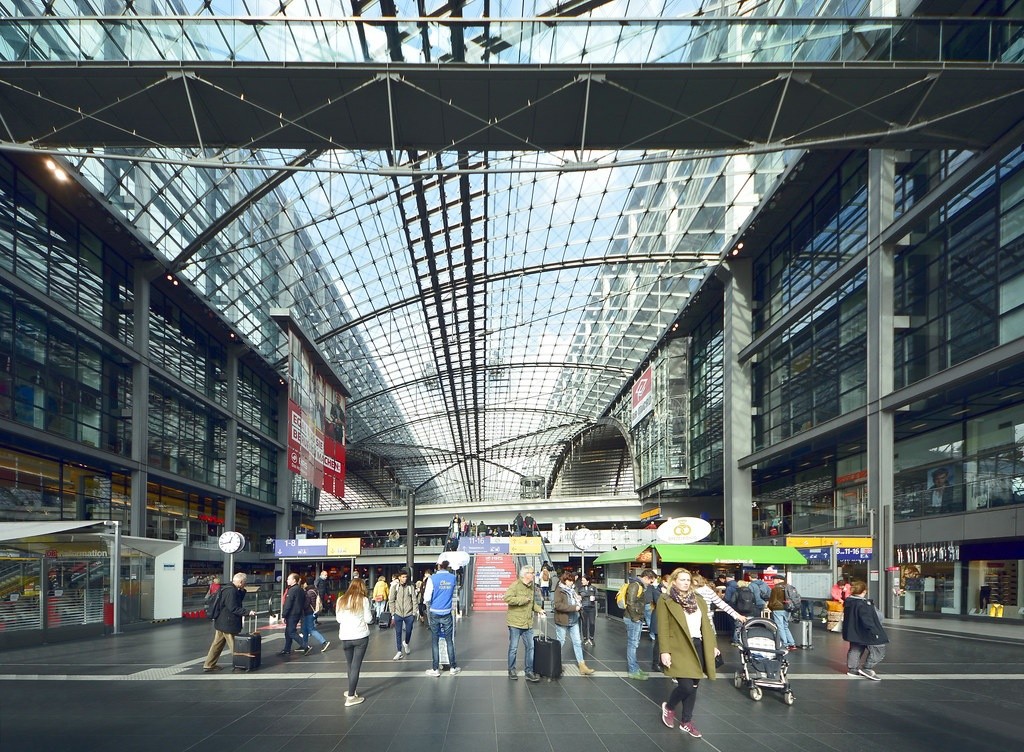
<box><xmin>572</xmin><ymin>528</ymin><xmax>594</xmax><ymax>550</ymax></box>
<box><xmin>219</xmin><ymin>531</ymin><xmax>245</xmax><ymax>553</ymax></box>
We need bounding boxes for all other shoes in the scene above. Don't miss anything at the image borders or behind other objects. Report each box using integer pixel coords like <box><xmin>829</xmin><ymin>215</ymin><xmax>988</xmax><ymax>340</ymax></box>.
<box><xmin>652</xmin><ymin>663</ymin><xmax>663</xmax><ymax>673</ymax></box>
<box><xmin>671</xmin><ymin>678</ymin><xmax>678</xmax><ymax>683</ymax></box>
<box><xmin>786</xmin><ymin>645</ymin><xmax>797</xmax><ymax>650</ymax></box>
<box><xmin>203</xmin><ymin>666</ymin><xmax>223</xmax><ymax>672</ymax></box>
<box><xmin>628</xmin><ymin>668</ymin><xmax>650</xmax><ymax>680</ymax></box>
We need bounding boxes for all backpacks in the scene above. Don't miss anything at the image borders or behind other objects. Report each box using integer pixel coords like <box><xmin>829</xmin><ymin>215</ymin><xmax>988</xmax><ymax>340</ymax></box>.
<box><xmin>736</xmin><ymin>586</ymin><xmax>754</xmax><ymax>613</ymax></box>
<box><xmin>205</xmin><ymin>584</ymin><xmax>235</xmax><ymax>618</ymax></box>
<box><xmin>307</xmin><ymin>590</ymin><xmax>323</xmax><ymax>615</ymax></box>
<box><xmin>616</xmin><ymin>581</ymin><xmax>643</xmax><ymax>608</ymax></box>
<box><xmin>777</xmin><ymin>584</ymin><xmax>802</xmax><ymax>611</ymax></box>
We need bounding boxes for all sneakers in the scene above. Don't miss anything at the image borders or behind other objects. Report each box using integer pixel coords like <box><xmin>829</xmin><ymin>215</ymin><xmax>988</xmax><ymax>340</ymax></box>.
<box><xmin>583</xmin><ymin>638</ymin><xmax>595</xmax><ymax>646</ymax></box>
<box><xmin>662</xmin><ymin>702</ymin><xmax>674</xmax><ymax>728</ymax></box>
<box><xmin>848</xmin><ymin>668</ymin><xmax>863</xmax><ymax>677</ymax></box>
<box><xmin>857</xmin><ymin>667</ymin><xmax>881</xmax><ymax>680</ymax></box>
<box><xmin>274</xmin><ymin>640</ymin><xmax>331</xmax><ymax>656</ymax></box>
<box><xmin>509</xmin><ymin>670</ymin><xmax>518</xmax><ymax>680</ymax></box>
<box><xmin>525</xmin><ymin>672</ymin><xmax>539</xmax><ymax>681</ymax></box>
<box><xmin>425</xmin><ymin>668</ymin><xmax>440</xmax><ymax>677</ymax></box>
<box><xmin>394</xmin><ymin>640</ymin><xmax>411</xmax><ymax>660</ymax></box>
<box><xmin>450</xmin><ymin>667</ymin><xmax>462</xmax><ymax>675</ymax></box>
<box><xmin>679</xmin><ymin>719</ymin><xmax>702</xmax><ymax>738</ymax></box>
<box><xmin>343</xmin><ymin>690</ymin><xmax>365</xmax><ymax>707</ymax></box>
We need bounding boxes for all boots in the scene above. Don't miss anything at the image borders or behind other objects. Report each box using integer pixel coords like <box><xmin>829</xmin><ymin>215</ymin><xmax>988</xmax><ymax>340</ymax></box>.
<box><xmin>578</xmin><ymin>661</ymin><xmax>595</xmax><ymax>675</ymax></box>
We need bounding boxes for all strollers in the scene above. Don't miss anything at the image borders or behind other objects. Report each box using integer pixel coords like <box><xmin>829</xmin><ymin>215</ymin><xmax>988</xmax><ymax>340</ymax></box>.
<box><xmin>731</xmin><ymin>616</ymin><xmax>796</xmax><ymax>705</ymax></box>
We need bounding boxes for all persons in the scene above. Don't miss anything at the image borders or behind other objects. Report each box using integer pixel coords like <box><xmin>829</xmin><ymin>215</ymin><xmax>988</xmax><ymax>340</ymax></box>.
<box><xmin>424</xmin><ymin>554</ymin><xmax>462</xmax><ymax>677</ymax></box>
<box><xmin>503</xmin><ymin>564</ymin><xmax>546</xmax><ymax>682</ymax></box>
<box><xmin>654</xmin><ymin>566</ymin><xmax>720</xmax><ymax>737</ymax></box>
<box><xmin>322</xmin><ymin>511</ymin><xmax>538</xmax><ymax>551</ymax></box>
<box><xmin>622</xmin><ymin>569</ymin><xmax>670</xmax><ymax>680</ymax></box>
<box><xmin>335</xmin><ymin>577</ymin><xmax>372</xmax><ymax>706</ymax></box>
<box><xmin>978</xmin><ymin>584</ymin><xmax>991</xmax><ymax>614</ymax></box>
<box><xmin>279</xmin><ymin>568</ymin><xmax>332</xmax><ymax>655</ymax></box>
<box><xmin>554</xmin><ymin>573</ymin><xmax>595</xmax><ymax>675</ymax></box>
<box><xmin>689</xmin><ymin>569</ymin><xmax>890</xmax><ymax>682</ymax></box>
<box><xmin>202</xmin><ymin>573</ymin><xmax>256</xmax><ymax>674</ymax></box>
<box><xmin>536</xmin><ymin>559</ymin><xmax>597</xmax><ymax>646</ymax></box>
<box><xmin>928</xmin><ymin>467</ymin><xmax>952</xmax><ymax>507</ymax></box>
<box><xmin>388</xmin><ymin>570</ymin><xmax>418</xmax><ymax>660</ymax></box>
<box><xmin>353</xmin><ymin>567</ymin><xmax>437</xmax><ymax>627</ymax></box>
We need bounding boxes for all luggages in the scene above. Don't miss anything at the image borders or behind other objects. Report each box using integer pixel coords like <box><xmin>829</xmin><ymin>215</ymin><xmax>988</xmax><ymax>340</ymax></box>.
<box><xmin>788</xmin><ymin>608</ymin><xmax>813</xmax><ymax>650</ymax></box>
<box><xmin>437</xmin><ymin>610</ymin><xmax>456</xmax><ymax>669</ymax></box>
<box><xmin>379</xmin><ymin>602</ymin><xmax>391</xmax><ymax>628</ymax></box>
<box><xmin>232</xmin><ymin>614</ymin><xmax>261</xmax><ymax>672</ymax></box>
<box><xmin>368</xmin><ymin>599</ymin><xmax>377</xmax><ymax>624</ymax></box>
<box><xmin>532</xmin><ymin>613</ymin><xmax>562</xmax><ymax>682</ymax></box>
